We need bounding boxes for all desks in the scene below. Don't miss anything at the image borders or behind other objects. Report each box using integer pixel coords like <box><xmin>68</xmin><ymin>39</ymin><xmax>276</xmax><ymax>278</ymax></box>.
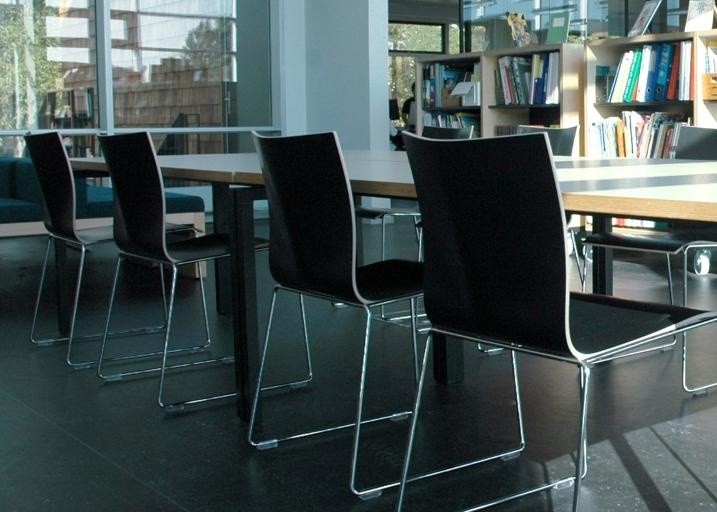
<box><xmin>68</xmin><ymin>149</ymin><xmax>716</xmax><ymax>422</ymax></box>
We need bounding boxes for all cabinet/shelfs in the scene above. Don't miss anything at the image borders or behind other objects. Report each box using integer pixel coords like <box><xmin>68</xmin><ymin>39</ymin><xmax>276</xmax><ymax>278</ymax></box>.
<box><xmin>413</xmin><ymin>44</ymin><xmax>584</xmax><ymax>156</ymax></box>
<box><xmin>584</xmin><ymin>30</ymin><xmax>717</xmax><ymax>159</ymax></box>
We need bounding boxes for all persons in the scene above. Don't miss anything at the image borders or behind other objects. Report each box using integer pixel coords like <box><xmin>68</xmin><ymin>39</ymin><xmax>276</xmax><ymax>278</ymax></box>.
<box><xmin>402</xmin><ymin>82</ymin><xmax>414</xmax><ymax>125</ymax></box>
<box><xmin>389</xmin><ymin>119</ymin><xmax>398</xmax><ymax>151</ymax></box>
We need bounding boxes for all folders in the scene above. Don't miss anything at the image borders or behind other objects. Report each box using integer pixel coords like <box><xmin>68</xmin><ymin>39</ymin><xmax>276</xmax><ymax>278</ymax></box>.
<box><xmin>636</xmin><ymin>43</ymin><xmax>674</xmax><ymax>103</ymax></box>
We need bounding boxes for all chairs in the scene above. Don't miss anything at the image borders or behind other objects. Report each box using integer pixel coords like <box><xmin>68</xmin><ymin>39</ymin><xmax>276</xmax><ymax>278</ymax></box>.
<box><xmin>21</xmin><ymin>132</ymin><xmax>211</xmax><ymax>368</ymax></box>
<box><xmin>97</xmin><ymin>129</ymin><xmax>311</xmax><ymax>411</ymax></box>
<box><xmin>330</xmin><ymin>126</ymin><xmax>474</xmax><ymax>321</ymax></box>
<box><xmin>579</xmin><ymin>123</ymin><xmax>717</xmax><ymax>398</ymax></box>
<box><xmin>245</xmin><ymin>131</ymin><xmax>525</xmax><ymax>495</ymax></box>
<box><xmin>518</xmin><ymin>124</ymin><xmax>584</xmax><ymax>288</ymax></box>
<box><xmin>394</xmin><ymin>131</ymin><xmax>717</xmax><ymax>512</ymax></box>
